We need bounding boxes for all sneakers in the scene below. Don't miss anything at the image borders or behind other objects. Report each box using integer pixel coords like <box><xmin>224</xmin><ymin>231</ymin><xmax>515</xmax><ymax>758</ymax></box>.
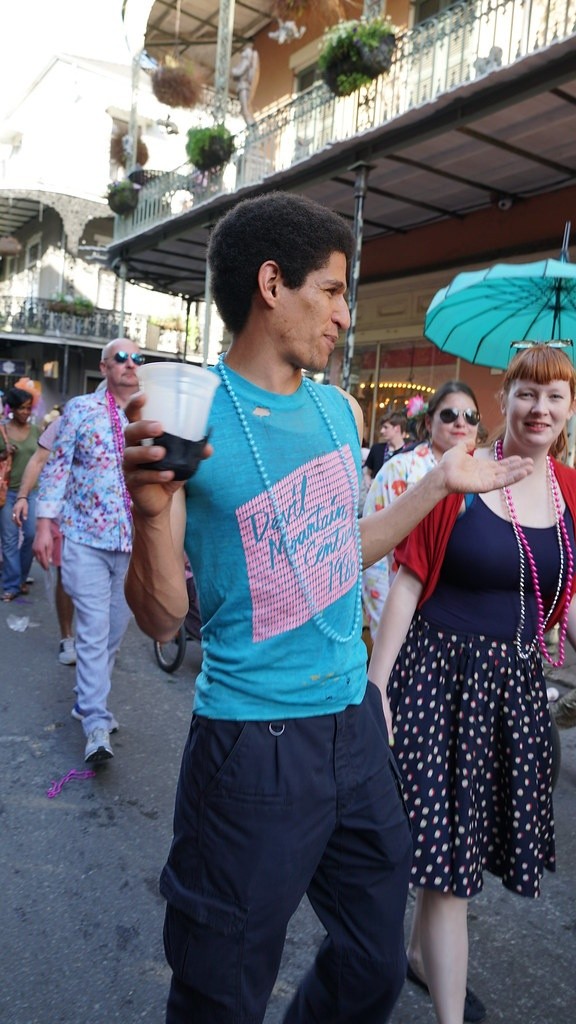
<box><xmin>85</xmin><ymin>729</ymin><xmax>114</xmax><ymax>762</ymax></box>
<box><xmin>72</xmin><ymin>703</ymin><xmax>120</xmax><ymax>733</ymax></box>
<box><xmin>60</xmin><ymin>635</ymin><xmax>77</xmax><ymax>664</ymax></box>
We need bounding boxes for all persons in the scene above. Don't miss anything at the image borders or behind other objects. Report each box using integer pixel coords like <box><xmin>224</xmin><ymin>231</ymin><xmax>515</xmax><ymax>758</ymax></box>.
<box><xmin>367</xmin><ymin>347</ymin><xmax>576</xmax><ymax>1024</ymax></box>
<box><xmin>11</xmin><ymin>380</ymin><xmax>108</xmax><ymax>664</ymax></box>
<box><xmin>122</xmin><ymin>194</ymin><xmax>532</xmax><ymax>1024</ymax></box>
<box><xmin>363</xmin><ymin>413</ymin><xmax>413</xmax><ymax>491</ymax></box>
<box><xmin>0</xmin><ymin>378</ymin><xmax>42</xmax><ymax>604</ymax></box>
<box><xmin>269</xmin><ymin>22</ymin><xmax>306</xmax><ymax>45</ymax></box>
<box><xmin>361</xmin><ymin>382</ymin><xmax>481</xmax><ymax>640</ymax></box>
<box><xmin>34</xmin><ymin>338</ymin><xmax>133</xmax><ymax>761</ymax></box>
<box><xmin>232</xmin><ymin>43</ymin><xmax>257</xmax><ymax>133</ymax></box>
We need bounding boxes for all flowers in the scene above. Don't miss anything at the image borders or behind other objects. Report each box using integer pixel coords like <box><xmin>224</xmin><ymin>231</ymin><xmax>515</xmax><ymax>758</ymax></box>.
<box><xmin>406</xmin><ymin>396</ymin><xmax>429</xmax><ymax>419</ymax></box>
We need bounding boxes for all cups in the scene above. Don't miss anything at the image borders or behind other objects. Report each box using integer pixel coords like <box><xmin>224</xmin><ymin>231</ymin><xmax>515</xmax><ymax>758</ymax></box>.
<box><xmin>133</xmin><ymin>361</ymin><xmax>221</xmax><ymax>481</ymax></box>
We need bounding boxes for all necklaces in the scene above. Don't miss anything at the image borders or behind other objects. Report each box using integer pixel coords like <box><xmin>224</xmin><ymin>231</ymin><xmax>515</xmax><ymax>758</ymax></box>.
<box><xmin>218</xmin><ymin>353</ymin><xmax>364</xmax><ymax>642</ymax></box>
<box><xmin>106</xmin><ymin>390</ymin><xmax>132</xmax><ymax>522</ymax></box>
<box><xmin>495</xmin><ymin>440</ymin><xmax>572</xmax><ymax>667</ymax></box>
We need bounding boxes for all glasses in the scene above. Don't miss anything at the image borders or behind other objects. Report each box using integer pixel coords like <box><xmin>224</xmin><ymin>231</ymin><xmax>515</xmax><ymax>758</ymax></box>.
<box><xmin>508</xmin><ymin>339</ymin><xmax>575</xmax><ymax>370</ymax></box>
<box><xmin>104</xmin><ymin>352</ymin><xmax>146</xmax><ymax>366</ymax></box>
<box><xmin>439</xmin><ymin>407</ymin><xmax>481</xmax><ymax>426</ymax></box>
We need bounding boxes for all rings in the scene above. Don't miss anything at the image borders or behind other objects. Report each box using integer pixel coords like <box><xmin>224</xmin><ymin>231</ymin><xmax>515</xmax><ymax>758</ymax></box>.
<box><xmin>12</xmin><ymin>513</ymin><xmax>16</xmax><ymax>517</ymax></box>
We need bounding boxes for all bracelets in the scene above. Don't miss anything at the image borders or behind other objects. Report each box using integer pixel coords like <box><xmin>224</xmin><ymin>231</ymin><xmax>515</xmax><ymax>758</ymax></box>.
<box><xmin>14</xmin><ymin>497</ymin><xmax>28</xmax><ymax>503</ymax></box>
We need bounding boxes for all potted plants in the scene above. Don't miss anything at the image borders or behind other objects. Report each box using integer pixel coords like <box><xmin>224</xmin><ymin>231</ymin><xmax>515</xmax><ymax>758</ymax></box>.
<box><xmin>51</xmin><ymin>294</ymin><xmax>95</xmax><ymax>317</ymax></box>
<box><xmin>102</xmin><ymin>181</ymin><xmax>138</xmax><ymax>212</ymax></box>
<box><xmin>319</xmin><ymin>14</ymin><xmax>401</xmax><ymax>97</ymax></box>
<box><xmin>185</xmin><ymin>120</ymin><xmax>235</xmax><ymax>176</ymax></box>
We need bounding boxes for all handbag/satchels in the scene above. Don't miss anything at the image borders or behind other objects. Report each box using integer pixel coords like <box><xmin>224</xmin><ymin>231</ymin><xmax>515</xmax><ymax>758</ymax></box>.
<box><xmin>0</xmin><ymin>426</ymin><xmax>12</xmax><ymax>506</ymax></box>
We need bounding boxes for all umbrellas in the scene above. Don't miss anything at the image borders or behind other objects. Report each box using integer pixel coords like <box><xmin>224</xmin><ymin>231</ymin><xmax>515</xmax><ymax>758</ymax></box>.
<box><xmin>423</xmin><ymin>223</ymin><xmax>576</xmax><ymax>371</ymax></box>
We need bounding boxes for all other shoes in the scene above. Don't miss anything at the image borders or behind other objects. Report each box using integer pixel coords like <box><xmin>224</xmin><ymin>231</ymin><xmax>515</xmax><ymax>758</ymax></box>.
<box><xmin>2</xmin><ymin>593</ymin><xmax>15</xmax><ymax>602</ymax></box>
<box><xmin>20</xmin><ymin>581</ymin><xmax>30</xmax><ymax>595</ymax></box>
<box><xmin>405</xmin><ymin>957</ymin><xmax>488</xmax><ymax>1022</ymax></box>
